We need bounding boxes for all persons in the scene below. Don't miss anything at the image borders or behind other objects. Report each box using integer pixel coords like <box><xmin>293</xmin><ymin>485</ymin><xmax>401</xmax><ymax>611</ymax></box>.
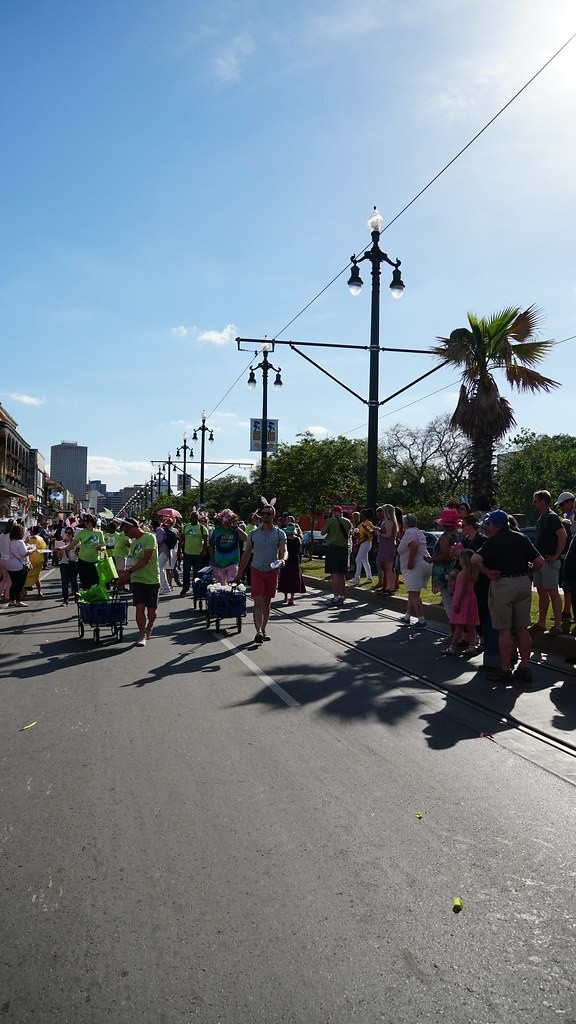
<box><xmin>278</xmin><ymin>516</ymin><xmax>306</xmax><ymax>606</ymax></box>
<box><xmin>0</xmin><ymin>514</ymin><xmax>133</xmax><ymax>606</ymax></box>
<box><xmin>113</xmin><ymin>517</ymin><xmax>160</xmax><ymax>646</ymax></box>
<box><xmin>424</xmin><ymin>495</ymin><xmax>545</xmax><ymax>682</ymax></box>
<box><xmin>139</xmin><ymin>509</ymin><xmax>262</xmax><ymax>595</ymax></box>
<box><xmin>397</xmin><ymin>514</ymin><xmax>433</xmax><ymax>629</ymax></box>
<box><xmin>233</xmin><ymin>505</ymin><xmax>288</xmax><ymax>643</ymax></box>
<box><xmin>527</xmin><ymin>490</ymin><xmax>576</xmax><ymax>635</ymax></box>
<box><xmin>321</xmin><ymin>505</ymin><xmax>403</xmax><ymax>606</ymax></box>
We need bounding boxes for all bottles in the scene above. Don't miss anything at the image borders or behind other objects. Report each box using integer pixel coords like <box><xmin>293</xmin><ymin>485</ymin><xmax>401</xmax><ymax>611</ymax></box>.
<box><xmin>97</xmin><ymin>550</ymin><xmax>105</xmax><ymax>561</ymax></box>
<box><xmin>270</xmin><ymin>561</ymin><xmax>282</xmax><ymax>569</ymax></box>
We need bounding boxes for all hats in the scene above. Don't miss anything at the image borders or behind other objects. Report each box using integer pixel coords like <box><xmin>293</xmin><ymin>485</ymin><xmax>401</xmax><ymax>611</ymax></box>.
<box><xmin>485</xmin><ymin>510</ymin><xmax>509</xmax><ymax>522</ymax></box>
<box><xmin>446</xmin><ymin>500</ymin><xmax>464</xmax><ymax>511</ymax></box>
<box><xmin>329</xmin><ymin>507</ymin><xmax>343</xmax><ymax>514</ymax></box>
<box><xmin>434</xmin><ymin>508</ymin><xmax>464</xmax><ymax>526</ymax></box>
<box><xmin>283</xmin><ymin>524</ymin><xmax>298</xmax><ymax>535</ymax></box>
<box><xmin>554</xmin><ymin>491</ymin><xmax>574</xmax><ymax>505</ymax></box>
<box><xmin>213</xmin><ymin>508</ymin><xmax>237</xmax><ymax>523</ymax></box>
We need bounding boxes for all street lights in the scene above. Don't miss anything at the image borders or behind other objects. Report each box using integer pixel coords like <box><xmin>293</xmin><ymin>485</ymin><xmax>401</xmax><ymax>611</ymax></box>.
<box><xmin>420</xmin><ymin>475</ymin><xmax>427</xmax><ymax>505</ymax></box>
<box><xmin>191</xmin><ymin>408</ymin><xmax>214</xmax><ymax>504</ymax></box>
<box><xmin>439</xmin><ymin>474</ymin><xmax>446</xmax><ymax>495</ymax></box>
<box><xmin>114</xmin><ymin>473</ymin><xmax>157</xmax><ymax>519</ymax></box>
<box><xmin>154</xmin><ymin>464</ymin><xmax>167</xmax><ymax>498</ymax></box>
<box><xmin>177</xmin><ymin>431</ymin><xmax>191</xmax><ymax>500</ymax></box>
<box><xmin>402</xmin><ymin>478</ymin><xmax>408</xmax><ymax>507</ymax></box>
<box><xmin>347</xmin><ymin>206</ymin><xmax>406</xmax><ymax>526</ymax></box>
<box><xmin>247</xmin><ymin>334</ymin><xmax>283</xmax><ymax>521</ymax></box>
<box><xmin>387</xmin><ymin>481</ymin><xmax>392</xmax><ymax>504</ymax></box>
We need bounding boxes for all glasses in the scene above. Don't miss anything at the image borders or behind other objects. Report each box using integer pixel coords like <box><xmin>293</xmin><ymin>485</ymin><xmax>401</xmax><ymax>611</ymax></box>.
<box><xmin>83</xmin><ymin>519</ymin><xmax>90</xmax><ymax>522</ymax></box>
<box><xmin>461</xmin><ymin>522</ymin><xmax>471</xmax><ymax>528</ymax></box>
<box><xmin>261</xmin><ymin>512</ymin><xmax>274</xmax><ymax>516</ymax></box>
<box><xmin>65</xmin><ymin>530</ymin><xmax>73</xmax><ymax>534</ymax></box>
<box><xmin>532</xmin><ymin>500</ymin><xmax>541</xmax><ymax>503</ymax></box>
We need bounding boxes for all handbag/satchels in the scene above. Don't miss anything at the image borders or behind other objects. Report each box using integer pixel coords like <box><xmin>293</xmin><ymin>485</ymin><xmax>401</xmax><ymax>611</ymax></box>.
<box><xmin>94</xmin><ymin>550</ymin><xmax>119</xmax><ymax>583</ymax></box>
<box><xmin>23</xmin><ymin>563</ymin><xmax>33</xmax><ymax>572</ymax></box>
<box><xmin>68</xmin><ymin>560</ymin><xmax>80</xmax><ymax>575</ymax></box>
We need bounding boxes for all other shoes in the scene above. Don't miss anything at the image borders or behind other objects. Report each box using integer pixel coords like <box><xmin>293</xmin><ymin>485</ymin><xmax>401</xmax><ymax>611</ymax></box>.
<box><xmin>487</xmin><ymin>669</ymin><xmax>514</xmax><ymax>681</ymax></box>
<box><xmin>551</xmin><ymin>611</ymin><xmax>573</xmax><ymax>621</ymax></box>
<box><xmin>569</xmin><ymin>627</ymin><xmax>576</xmax><ymax>639</ymax></box>
<box><xmin>562</xmin><ymin>621</ymin><xmax>571</xmax><ymax>636</ymax></box>
<box><xmin>510</xmin><ymin>664</ymin><xmax>532</xmax><ymax>683</ymax></box>
<box><xmin>545</xmin><ymin>627</ymin><xmax>562</xmax><ymax>638</ymax></box>
<box><xmin>527</xmin><ymin>623</ymin><xmax>547</xmax><ymax>633</ymax></box>
<box><xmin>0</xmin><ymin>555</ymin><xmax>482</xmax><ymax>659</ymax></box>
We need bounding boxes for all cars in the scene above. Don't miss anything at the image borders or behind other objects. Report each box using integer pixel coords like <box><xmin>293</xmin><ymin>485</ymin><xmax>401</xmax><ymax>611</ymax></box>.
<box><xmin>303</xmin><ymin>531</ymin><xmax>328</xmax><ymax>559</ymax></box>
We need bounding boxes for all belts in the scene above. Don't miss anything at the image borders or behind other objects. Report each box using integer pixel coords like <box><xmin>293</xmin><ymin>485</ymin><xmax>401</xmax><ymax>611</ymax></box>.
<box><xmin>500</xmin><ymin>573</ymin><xmax>527</xmax><ymax>577</ymax></box>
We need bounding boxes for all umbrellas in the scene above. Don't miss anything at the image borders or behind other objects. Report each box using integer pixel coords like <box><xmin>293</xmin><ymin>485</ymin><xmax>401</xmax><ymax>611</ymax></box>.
<box><xmin>157</xmin><ymin>508</ymin><xmax>182</xmax><ymax>518</ymax></box>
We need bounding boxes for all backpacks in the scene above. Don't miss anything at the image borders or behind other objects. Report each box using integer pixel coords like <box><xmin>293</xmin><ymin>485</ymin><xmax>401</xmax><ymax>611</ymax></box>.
<box><xmin>163</xmin><ymin>528</ymin><xmax>178</xmax><ymax>550</ymax></box>
